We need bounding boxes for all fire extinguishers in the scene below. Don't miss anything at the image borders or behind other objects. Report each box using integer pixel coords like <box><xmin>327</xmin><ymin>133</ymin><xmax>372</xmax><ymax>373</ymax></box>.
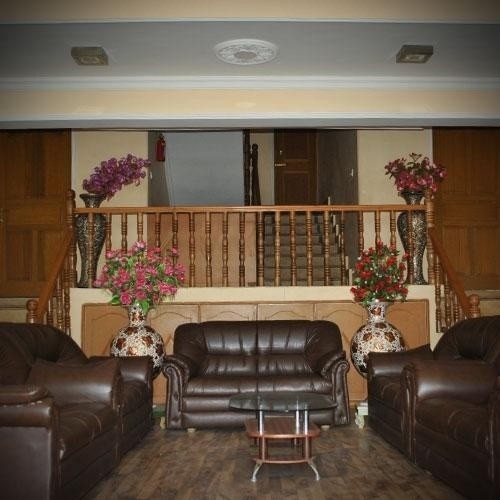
<box><xmin>155</xmin><ymin>133</ymin><xmax>166</xmax><ymax>161</ymax></box>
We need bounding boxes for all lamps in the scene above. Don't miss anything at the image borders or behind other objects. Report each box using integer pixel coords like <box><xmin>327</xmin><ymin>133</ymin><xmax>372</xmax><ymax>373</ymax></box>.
<box><xmin>397</xmin><ymin>45</ymin><xmax>433</xmax><ymax>64</ymax></box>
<box><xmin>71</xmin><ymin>47</ymin><xmax>108</xmax><ymax>66</ymax></box>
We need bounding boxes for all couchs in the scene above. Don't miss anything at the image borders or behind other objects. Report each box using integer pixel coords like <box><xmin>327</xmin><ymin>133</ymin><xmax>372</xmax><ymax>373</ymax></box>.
<box><xmin>161</xmin><ymin>320</ymin><xmax>350</xmax><ymax>433</ymax></box>
<box><xmin>368</xmin><ymin>315</ymin><xmax>500</xmax><ymax>500</ymax></box>
<box><xmin>0</xmin><ymin>321</ymin><xmax>153</xmax><ymax>500</ymax></box>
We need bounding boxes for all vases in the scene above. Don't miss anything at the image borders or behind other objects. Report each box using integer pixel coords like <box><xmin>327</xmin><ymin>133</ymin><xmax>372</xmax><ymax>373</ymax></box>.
<box><xmin>75</xmin><ymin>193</ymin><xmax>106</xmax><ymax>287</ymax></box>
<box><xmin>109</xmin><ymin>305</ymin><xmax>167</xmax><ymax>379</ymax></box>
<box><xmin>397</xmin><ymin>190</ymin><xmax>426</xmax><ymax>285</ymax></box>
<box><xmin>351</xmin><ymin>303</ymin><xmax>405</xmax><ymax>407</ymax></box>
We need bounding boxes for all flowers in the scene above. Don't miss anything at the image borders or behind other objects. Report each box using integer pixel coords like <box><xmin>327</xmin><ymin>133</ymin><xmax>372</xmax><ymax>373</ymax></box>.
<box><xmin>349</xmin><ymin>239</ymin><xmax>411</xmax><ymax>306</ymax></box>
<box><xmin>93</xmin><ymin>239</ymin><xmax>185</xmax><ymax>315</ymax></box>
<box><xmin>384</xmin><ymin>151</ymin><xmax>447</xmax><ymax>199</ymax></box>
<box><xmin>82</xmin><ymin>153</ymin><xmax>154</xmax><ymax>201</ymax></box>
